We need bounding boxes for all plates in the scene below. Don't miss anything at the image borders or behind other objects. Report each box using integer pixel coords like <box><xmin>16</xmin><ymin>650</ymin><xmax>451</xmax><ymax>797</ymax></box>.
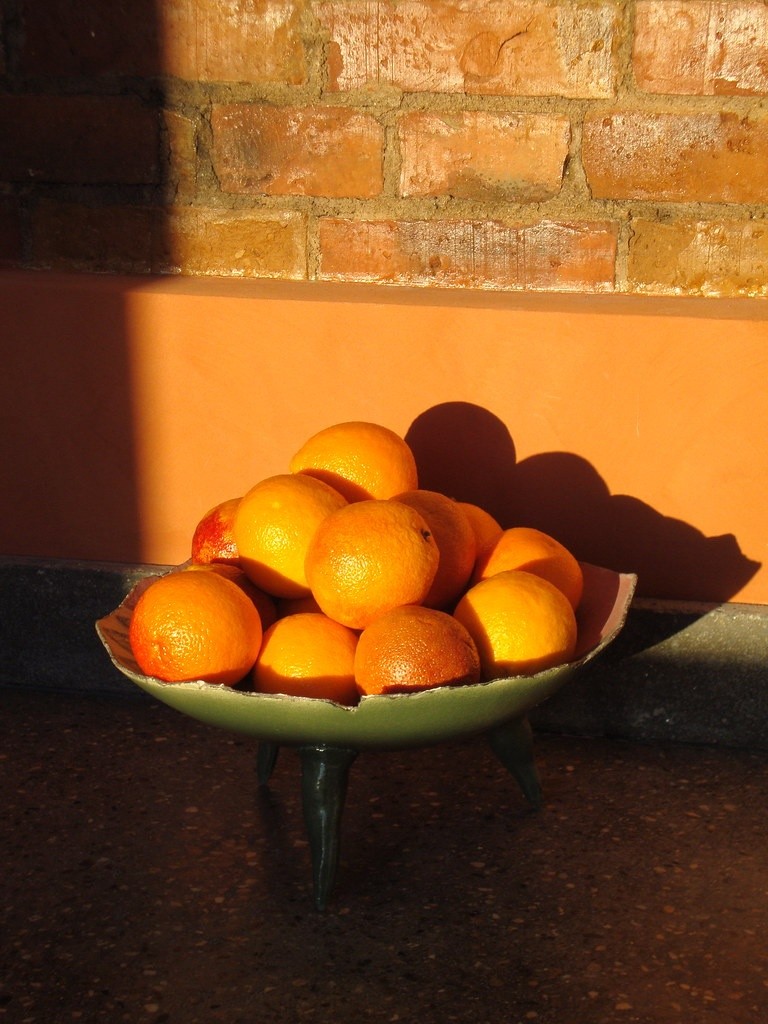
<box><xmin>92</xmin><ymin>561</ymin><xmax>638</xmax><ymax>913</ymax></box>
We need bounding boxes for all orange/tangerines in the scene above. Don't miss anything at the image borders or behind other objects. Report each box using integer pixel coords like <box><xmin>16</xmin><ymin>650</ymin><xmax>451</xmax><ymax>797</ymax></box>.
<box><xmin>129</xmin><ymin>422</ymin><xmax>584</xmax><ymax>706</ymax></box>
